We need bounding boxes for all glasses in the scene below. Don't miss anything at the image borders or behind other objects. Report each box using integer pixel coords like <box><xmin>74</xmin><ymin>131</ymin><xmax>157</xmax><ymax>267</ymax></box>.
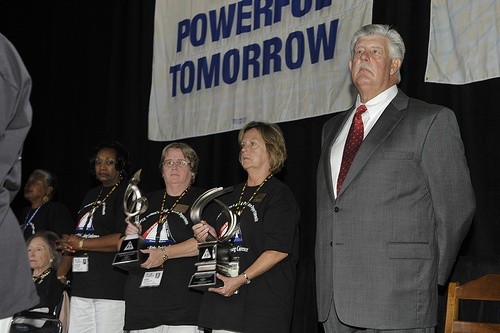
<box><xmin>162</xmin><ymin>160</ymin><xmax>196</xmax><ymax>172</ymax></box>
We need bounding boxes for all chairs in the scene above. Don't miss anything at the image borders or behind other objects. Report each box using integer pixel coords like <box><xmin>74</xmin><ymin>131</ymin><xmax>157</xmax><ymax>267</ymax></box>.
<box><xmin>444</xmin><ymin>272</ymin><xmax>500</xmax><ymax>333</ymax></box>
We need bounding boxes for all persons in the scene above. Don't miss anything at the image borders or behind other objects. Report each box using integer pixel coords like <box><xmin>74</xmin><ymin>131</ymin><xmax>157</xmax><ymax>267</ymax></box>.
<box><xmin>119</xmin><ymin>141</ymin><xmax>213</xmax><ymax>333</ymax></box>
<box><xmin>192</xmin><ymin>120</ymin><xmax>300</xmax><ymax>333</ymax></box>
<box><xmin>0</xmin><ymin>31</ymin><xmax>40</xmax><ymax>333</ymax></box>
<box><xmin>313</xmin><ymin>24</ymin><xmax>476</xmax><ymax>333</ymax></box>
<box><xmin>10</xmin><ymin>168</ymin><xmax>71</xmax><ymax>333</ymax></box>
<box><xmin>56</xmin><ymin>142</ymin><xmax>144</xmax><ymax>333</ymax></box>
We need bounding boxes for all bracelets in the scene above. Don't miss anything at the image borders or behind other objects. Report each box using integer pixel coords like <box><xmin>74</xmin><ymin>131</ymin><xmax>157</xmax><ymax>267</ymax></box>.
<box><xmin>242</xmin><ymin>273</ymin><xmax>251</xmax><ymax>284</ymax></box>
<box><xmin>161</xmin><ymin>247</ymin><xmax>168</xmax><ymax>261</ymax></box>
<box><xmin>57</xmin><ymin>275</ymin><xmax>65</xmax><ymax>280</ymax></box>
<box><xmin>79</xmin><ymin>238</ymin><xmax>84</xmax><ymax>251</ymax></box>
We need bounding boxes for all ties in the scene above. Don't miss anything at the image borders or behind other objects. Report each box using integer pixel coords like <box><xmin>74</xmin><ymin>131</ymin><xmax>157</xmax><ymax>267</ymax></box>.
<box><xmin>336</xmin><ymin>105</ymin><xmax>367</xmax><ymax>198</ymax></box>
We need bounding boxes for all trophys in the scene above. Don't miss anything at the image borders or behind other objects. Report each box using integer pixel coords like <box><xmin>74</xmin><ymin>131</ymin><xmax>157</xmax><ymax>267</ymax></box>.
<box><xmin>112</xmin><ymin>169</ymin><xmax>151</xmax><ymax>272</ymax></box>
<box><xmin>188</xmin><ymin>187</ymin><xmax>238</xmax><ymax>291</ymax></box>
